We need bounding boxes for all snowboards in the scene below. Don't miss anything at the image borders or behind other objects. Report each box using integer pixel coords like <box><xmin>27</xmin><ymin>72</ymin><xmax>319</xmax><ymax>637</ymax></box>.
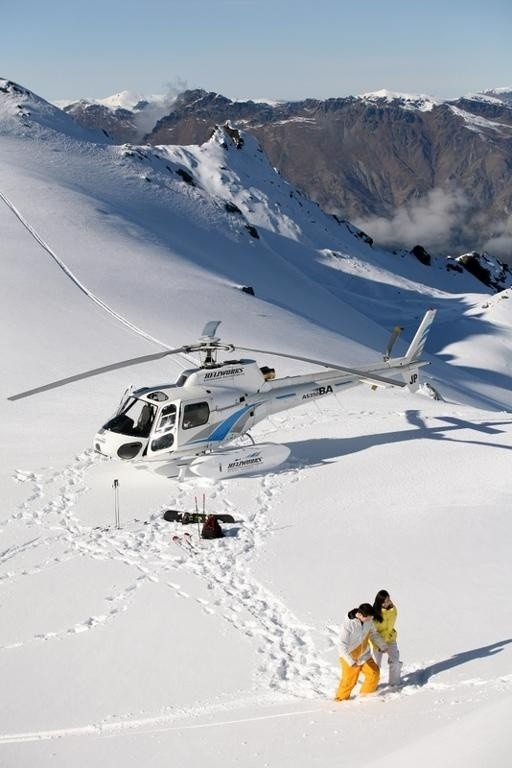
<box><xmin>163</xmin><ymin>511</ymin><xmax>234</xmax><ymax>524</ymax></box>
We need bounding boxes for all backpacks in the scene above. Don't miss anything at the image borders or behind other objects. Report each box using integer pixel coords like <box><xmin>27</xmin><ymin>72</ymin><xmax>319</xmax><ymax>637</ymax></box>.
<box><xmin>201</xmin><ymin>515</ymin><xmax>221</xmax><ymax>539</ymax></box>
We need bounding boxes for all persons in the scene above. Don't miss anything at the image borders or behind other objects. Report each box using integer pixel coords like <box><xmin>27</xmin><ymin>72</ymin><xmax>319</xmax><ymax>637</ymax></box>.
<box><xmin>372</xmin><ymin>590</ymin><xmax>403</xmax><ymax>688</ymax></box>
<box><xmin>335</xmin><ymin>603</ymin><xmax>388</xmax><ymax>702</ymax></box>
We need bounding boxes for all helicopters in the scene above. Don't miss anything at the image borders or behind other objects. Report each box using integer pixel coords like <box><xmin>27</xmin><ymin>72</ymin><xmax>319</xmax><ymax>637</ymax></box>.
<box><xmin>7</xmin><ymin>307</ymin><xmax>444</xmax><ymax>480</ymax></box>
